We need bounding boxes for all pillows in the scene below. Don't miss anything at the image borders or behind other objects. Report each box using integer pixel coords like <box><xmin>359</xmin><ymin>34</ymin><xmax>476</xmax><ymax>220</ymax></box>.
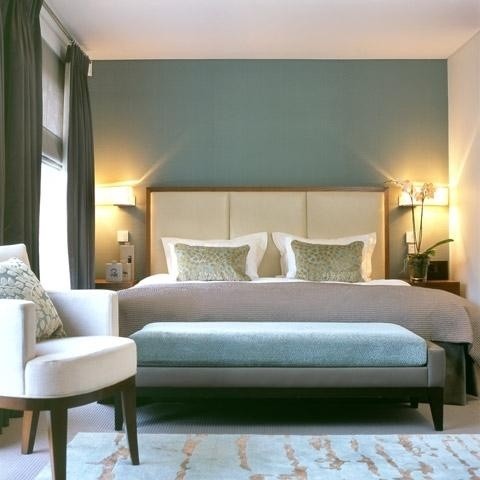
<box><xmin>157</xmin><ymin>231</ymin><xmax>378</xmax><ymax>283</ymax></box>
<box><xmin>0</xmin><ymin>258</ymin><xmax>66</xmax><ymax>343</ymax></box>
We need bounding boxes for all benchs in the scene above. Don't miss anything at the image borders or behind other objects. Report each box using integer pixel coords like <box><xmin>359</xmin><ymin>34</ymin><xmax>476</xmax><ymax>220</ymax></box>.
<box><xmin>115</xmin><ymin>321</ymin><xmax>447</xmax><ymax>432</ymax></box>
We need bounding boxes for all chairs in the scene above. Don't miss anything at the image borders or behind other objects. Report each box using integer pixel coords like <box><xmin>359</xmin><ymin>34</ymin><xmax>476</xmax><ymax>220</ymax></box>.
<box><xmin>0</xmin><ymin>242</ymin><xmax>138</xmax><ymax>480</ymax></box>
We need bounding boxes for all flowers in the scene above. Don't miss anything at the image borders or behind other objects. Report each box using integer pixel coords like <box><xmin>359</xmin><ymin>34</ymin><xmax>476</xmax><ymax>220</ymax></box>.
<box><xmin>384</xmin><ymin>176</ymin><xmax>453</xmax><ymax>278</ymax></box>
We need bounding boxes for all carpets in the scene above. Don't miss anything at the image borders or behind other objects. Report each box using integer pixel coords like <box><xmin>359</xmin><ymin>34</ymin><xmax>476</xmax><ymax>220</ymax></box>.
<box><xmin>35</xmin><ymin>430</ymin><xmax>480</xmax><ymax>479</ymax></box>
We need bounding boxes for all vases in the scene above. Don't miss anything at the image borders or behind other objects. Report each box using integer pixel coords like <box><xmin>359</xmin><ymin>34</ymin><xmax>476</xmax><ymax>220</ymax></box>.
<box><xmin>406</xmin><ymin>254</ymin><xmax>429</xmax><ymax>285</ymax></box>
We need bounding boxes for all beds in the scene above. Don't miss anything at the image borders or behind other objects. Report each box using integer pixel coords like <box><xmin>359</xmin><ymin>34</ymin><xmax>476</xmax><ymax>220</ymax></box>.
<box><xmin>100</xmin><ymin>187</ymin><xmax>479</xmax><ymax>404</ymax></box>
